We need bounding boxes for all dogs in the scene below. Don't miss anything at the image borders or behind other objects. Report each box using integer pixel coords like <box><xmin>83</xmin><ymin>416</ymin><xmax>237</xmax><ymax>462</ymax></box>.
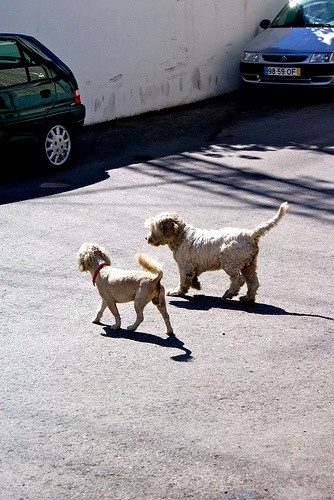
<box><xmin>77</xmin><ymin>241</ymin><xmax>175</xmax><ymax>335</ymax></box>
<box><xmin>143</xmin><ymin>201</ymin><xmax>289</xmax><ymax>305</ymax></box>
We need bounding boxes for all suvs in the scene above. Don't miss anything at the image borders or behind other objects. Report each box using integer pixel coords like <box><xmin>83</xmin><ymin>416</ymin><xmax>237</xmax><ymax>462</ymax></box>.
<box><xmin>0</xmin><ymin>32</ymin><xmax>87</xmax><ymax>170</ymax></box>
<box><xmin>238</xmin><ymin>0</ymin><xmax>334</xmax><ymax>86</ymax></box>
